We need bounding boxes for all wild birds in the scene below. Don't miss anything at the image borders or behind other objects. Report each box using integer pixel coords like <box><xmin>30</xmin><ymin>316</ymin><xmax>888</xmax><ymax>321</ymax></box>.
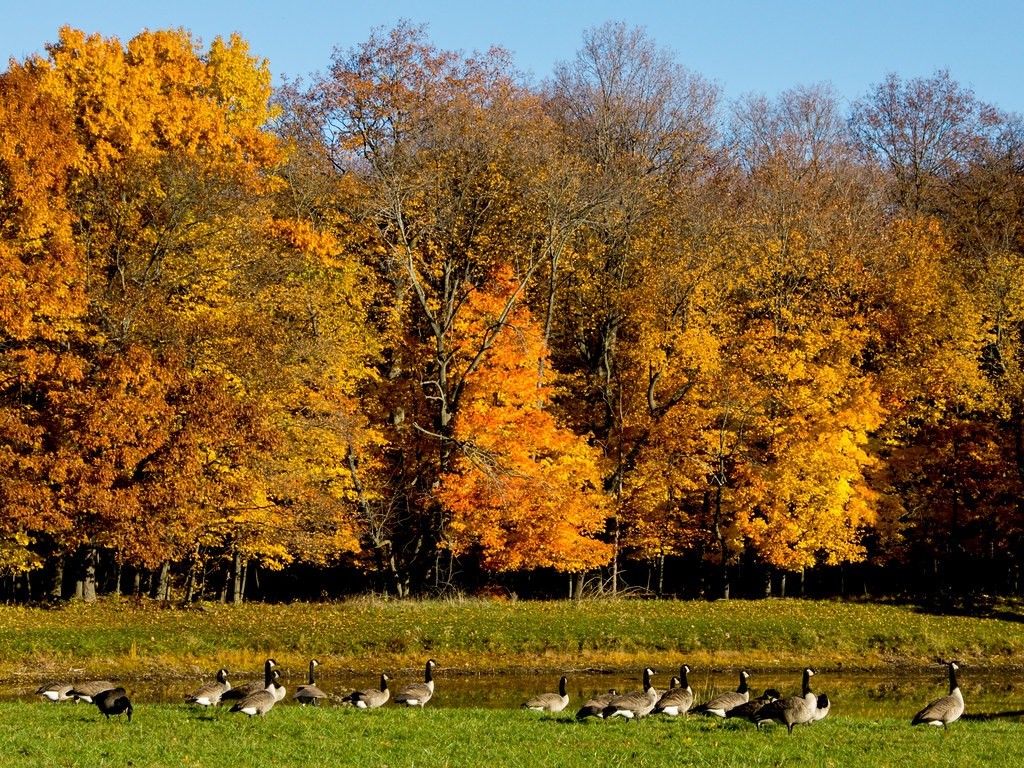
<box><xmin>518</xmin><ymin>663</ymin><xmax>831</xmax><ymax>735</ymax></box>
<box><xmin>35</xmin><ymin>681</ymin><xmax>133</xmax><ymax>722</ymax></box>
<box><xmin>911</xmin><ymin>661</ymin><xmax>964</xmax><ymax>732</ymax></box>
<box><xmin>395</xmin><ymin>659</ymin><xmax>441</xmax><ymax>709</ymax></box>
<box><xmin>184</xmin><ymin>669</ymin><xmax>231</xmax><ymax>707</ymax></box>
<box><xmin>342</xmin><ymin>673</ymin><xmax>390</xmax><ymax>709</ymax></box>
<box><xmin>220</xmin><ymin>659</ymin><xmax>286</xmax><ymax>718</ymax></box>
<box><xmin>292</xmin><ymin>659</ymin><xmax>327</xmax><ymax>706</ymax></box>
